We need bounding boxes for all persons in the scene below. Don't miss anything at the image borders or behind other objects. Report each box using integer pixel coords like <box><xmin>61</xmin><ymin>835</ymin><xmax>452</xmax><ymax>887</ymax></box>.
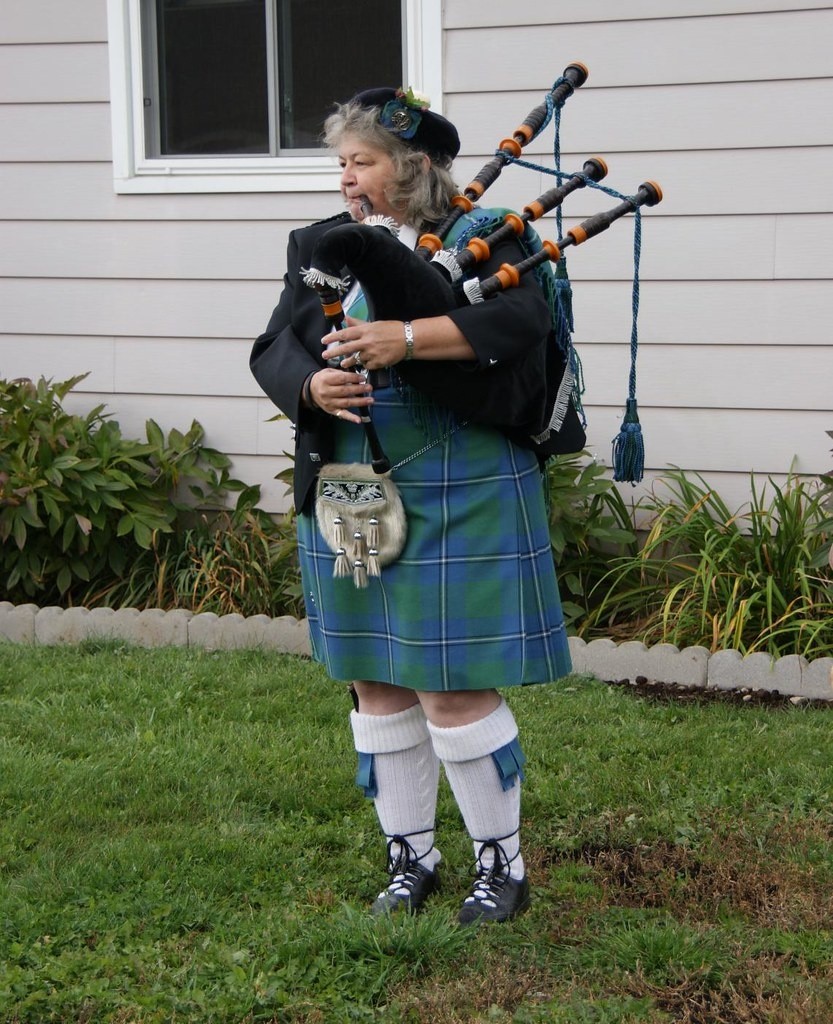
<box><xmin>249</xmin><ymin>87</ymin><xmax>589</xmax><ymax>925</ymax></box>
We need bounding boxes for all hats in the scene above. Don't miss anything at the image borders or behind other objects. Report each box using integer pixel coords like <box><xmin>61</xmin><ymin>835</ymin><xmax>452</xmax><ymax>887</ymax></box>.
<box><xmin>347</xmin><ymin>87</ymin><xmax>461</xmax><ymax>167</ymax></box>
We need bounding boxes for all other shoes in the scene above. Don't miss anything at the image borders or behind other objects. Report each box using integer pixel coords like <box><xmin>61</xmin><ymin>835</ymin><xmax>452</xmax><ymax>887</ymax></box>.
<box><xmin>369</xmin><ymin>828</ymin><xmax>441</xmax><ymax>917</ymax></box>
<box><xmin>457</xmin><ymin>828</ymin><xmax>531</xmax><ymax>928</ymax></box>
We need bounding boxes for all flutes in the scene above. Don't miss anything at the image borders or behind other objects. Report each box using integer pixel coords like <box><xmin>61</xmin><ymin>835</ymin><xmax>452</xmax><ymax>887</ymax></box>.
<box><xmin>299</xmin><ymin>57</ymin><xmax>666</xmax><ymax>476</ymax></box>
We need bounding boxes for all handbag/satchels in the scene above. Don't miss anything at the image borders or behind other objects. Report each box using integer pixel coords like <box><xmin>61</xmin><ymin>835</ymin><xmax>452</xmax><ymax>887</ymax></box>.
<box><xmin>316</xmin><ymin>461</ymin><xmax>407</xmax><ymax>588</ymax></box>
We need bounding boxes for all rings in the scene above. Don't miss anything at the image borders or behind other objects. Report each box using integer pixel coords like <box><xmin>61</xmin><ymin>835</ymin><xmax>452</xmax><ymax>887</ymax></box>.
<box><xmin>336</xmin><ymin>410</ymin><xmax>345</xmax><ymax>418</ymax></box>
<box><xmin>355</xmin><ymin>351</ymin><xmax>362</xmax><ymax>364</ymax></box>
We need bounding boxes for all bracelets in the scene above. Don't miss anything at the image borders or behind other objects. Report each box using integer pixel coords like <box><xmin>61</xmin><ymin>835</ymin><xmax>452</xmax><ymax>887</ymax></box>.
<box><xmin>404</xmin><ymin>321</ymin><xmax>414</xmax><ymax>358</ymax></box>
